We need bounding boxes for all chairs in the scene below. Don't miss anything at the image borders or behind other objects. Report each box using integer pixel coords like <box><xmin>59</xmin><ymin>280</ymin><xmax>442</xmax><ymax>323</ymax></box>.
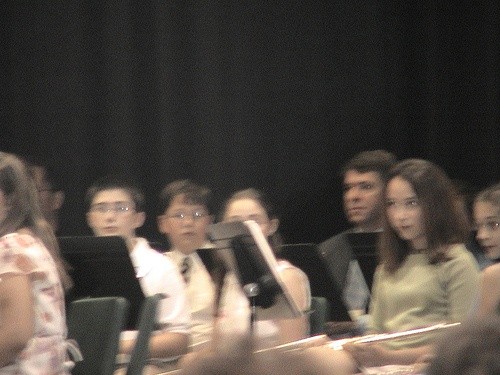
<box><xmin>66</xmin><ymin>296</ymin><xmax>126</xmax><ymax>375</ymax></box>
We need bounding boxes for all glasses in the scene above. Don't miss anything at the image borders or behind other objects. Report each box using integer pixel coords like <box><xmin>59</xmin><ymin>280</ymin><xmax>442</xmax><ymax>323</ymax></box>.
<box><xmin>166</xmin><ymin>212</ymin><xmax>210</xmax><ymax>219</ymax></box>
<box><xmin>89</xmin><ymin>207</ymin><xmax>136</xmax><ymax>213</ymax></box>
<box><xmin>473</xmin><ymin>223</ymin><xmax>500</xmax><ymax>230</ymax></box>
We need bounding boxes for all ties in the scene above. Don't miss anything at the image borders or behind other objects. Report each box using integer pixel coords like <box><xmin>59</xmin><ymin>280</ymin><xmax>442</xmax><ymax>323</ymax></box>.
<box><xmin>181</xmin><ymin>258</ymin><xmax>193</xmax><ymax>283</ymax></box>
